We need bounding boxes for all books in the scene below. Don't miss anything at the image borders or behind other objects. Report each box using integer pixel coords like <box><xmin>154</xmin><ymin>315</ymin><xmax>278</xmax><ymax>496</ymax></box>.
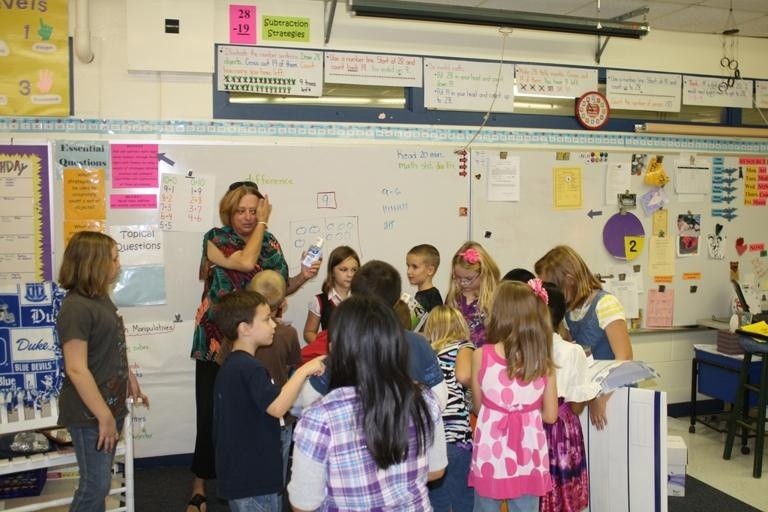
<box><xmin>47</xmin><ymin>463</ymin><xmax>116</xmax><ymax>479</ymax></box>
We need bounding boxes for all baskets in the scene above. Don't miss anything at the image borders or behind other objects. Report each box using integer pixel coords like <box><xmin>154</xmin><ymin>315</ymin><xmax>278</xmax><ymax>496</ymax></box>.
<box><xmin>0</xmin><ymin>466</ymin><xmax>46</xmax><ymax>497</ymax></box>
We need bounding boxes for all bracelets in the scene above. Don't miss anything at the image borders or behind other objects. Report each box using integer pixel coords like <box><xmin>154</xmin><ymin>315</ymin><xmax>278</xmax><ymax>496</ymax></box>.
<box><xmin>259</xmin><ymin>221</ymin><xmax>270</xmax><ymax>229</ymax></box>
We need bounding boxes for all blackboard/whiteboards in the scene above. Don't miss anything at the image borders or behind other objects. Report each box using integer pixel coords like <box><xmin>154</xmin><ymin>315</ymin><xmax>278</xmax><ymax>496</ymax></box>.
<box><xmin>471</xmin><ymin>146</ymin><xmax>767</xmax><ymax>335</ymax></box>
<box><xmin>48</xmin><ymin>142</ymin><xmax>471</xmax><ymax>376</ymax></box>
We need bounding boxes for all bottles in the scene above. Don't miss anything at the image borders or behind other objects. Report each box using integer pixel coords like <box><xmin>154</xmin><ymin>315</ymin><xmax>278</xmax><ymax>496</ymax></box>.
<box><xmin>131</xmin><ymin>398</ymin><xmax>151</xmax><ymax>440</ymax></box>
<box><xmin>729</xmin><ymin>311</ymin><xmax>739</xmax><ymax>335</ymax></box>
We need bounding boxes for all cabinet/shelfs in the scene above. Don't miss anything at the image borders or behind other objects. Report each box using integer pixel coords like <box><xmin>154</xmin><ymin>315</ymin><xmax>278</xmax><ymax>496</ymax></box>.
<box><xmin>1</xmin><ymin>396</ymin><xmax>144</xmax><ymax>511</ymax></box>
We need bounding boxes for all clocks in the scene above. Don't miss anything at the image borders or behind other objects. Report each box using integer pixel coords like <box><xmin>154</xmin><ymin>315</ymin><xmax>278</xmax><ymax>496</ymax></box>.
<box><xmin>576</xmin><ymin>91</ymin><xmax>611</xmax><ymax>130</ymax></box>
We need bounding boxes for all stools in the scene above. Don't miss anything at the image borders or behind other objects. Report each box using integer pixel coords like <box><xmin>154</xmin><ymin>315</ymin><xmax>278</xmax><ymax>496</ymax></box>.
<box><xmin>723</xmin><ymin>334</ymin><xmax>768</xmax><ymax>478</ymax></box>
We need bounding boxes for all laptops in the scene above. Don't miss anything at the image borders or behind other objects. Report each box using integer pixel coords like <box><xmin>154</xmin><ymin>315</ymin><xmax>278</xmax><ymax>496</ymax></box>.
<box><xmin>731</xmin><ymin>279</ymin><xmax>768</xmax><ymax>325</ymax></box>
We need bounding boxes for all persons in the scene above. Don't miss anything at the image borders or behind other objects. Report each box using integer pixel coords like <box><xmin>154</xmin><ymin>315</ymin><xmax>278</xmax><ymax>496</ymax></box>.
<box><xmin>535</xmin><ymin>243</ymin><xmax>626</xmax><ymax>511</ymax></box>
<box><xmin>299</xmin><ymin>298</ymin><xmax>412</xmax><ymax>369</ymax></box>
<box><xmin>417</xmin><ymin>305</ymin><xmax>477</xmax><ymax>512</ymax></box>
<box><xmin>186</xmin><ymin>181</ymin><xmax>323</xmax><ymax>511</ymax></box>
<box><xmin>213</xmin><ymin>290</ymin><xmax>329</xmax><ymax>511</ymax></box>
<box><xmin>406</xmin><ymin>244</ymin><xmax>444</xmax><ymax>331</ymax></box>
<box><xmin>290</xmin><ymin>260</ymin><xmax>449</xmax><ymax>417</ymax></box>
<box><xmin>540</xmin><ymin>280</ymin><xmax>601</xmax><ymax>512</ymax></box>
<box><xmin>445</xmin><ymin>241</ymin><xmax>502</xmax><ymax>349</ymax></box>
<box><xmin>466</xmin><ymin>279</ymin><xmax>560</xmax><ymax>511</ymax></box>
<box><xmin>303</xmin><ymin>245</ymin><xmax>362</xmax><ymax>343</ymax></box>
<box><xmin>503</xmin><ymin>268</ymin><xmax>536</xmax><ymax>283</ymax></box>
<box><xmin>203</xmin><ymin>269</ymin><xmax>301</xmax><ymax>489</ymax></box>
<box><xmin>286</xmin><ymin>295</ymin><xmax>450</xmax><ymax>512</ymax></box>
<box><xmin>56</xmin><ymin>232</ymin><xmax>150</xmax><ymax>512</ymax></box>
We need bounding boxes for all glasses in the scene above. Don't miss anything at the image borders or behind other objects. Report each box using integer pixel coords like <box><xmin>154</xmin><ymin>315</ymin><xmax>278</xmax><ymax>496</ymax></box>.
<box><xmin>228</xmin><ymin>182</ymin><xmax>258</xmax><ymax>193</ymax></box>
